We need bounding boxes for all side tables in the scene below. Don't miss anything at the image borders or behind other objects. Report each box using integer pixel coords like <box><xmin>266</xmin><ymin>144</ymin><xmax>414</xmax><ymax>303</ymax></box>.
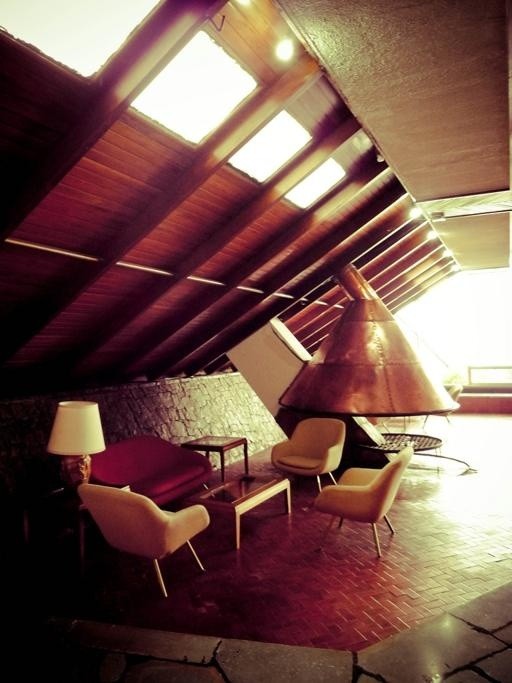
<box><xmin>181</xmin><ymin>435</ymin><xmax>248</xmax><ymax>474</ymax></box>
<box><xmin>16</xmin><ymin>480</ymin><xmax>132</xmax><ymax>576</ymax></box>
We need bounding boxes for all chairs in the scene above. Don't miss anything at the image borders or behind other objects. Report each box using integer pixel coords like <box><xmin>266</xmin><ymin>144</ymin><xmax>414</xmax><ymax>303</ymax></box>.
<box><xmin>314</xmin><ymin>447</ymin><xmax>415</xmax><ymax>559</ymax></box>
<box><xmin>60</xmin><ymin>434</ymin><xmax>213</xmax><ymax>507</ymax></box>
<box><xmin>271</xmin><ymin>418</ymin><xmax>347</xmax><ymax>494</ymax></box>
<box><xmin>422</xmin><ymin>383</ymin><xmax>464</xmax><ymax>429</ymax></box>
<box><xmin>77</xmin><ymin>484</ymin><xmax>211</xmax><ymax>598</ymax></box>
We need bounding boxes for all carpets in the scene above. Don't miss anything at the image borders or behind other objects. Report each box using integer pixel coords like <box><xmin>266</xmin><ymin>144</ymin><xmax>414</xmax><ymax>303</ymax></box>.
<box><xmin>0</xmin><ymin>581</ymin><xmax>512</xmax><ymax>683</ymax></box>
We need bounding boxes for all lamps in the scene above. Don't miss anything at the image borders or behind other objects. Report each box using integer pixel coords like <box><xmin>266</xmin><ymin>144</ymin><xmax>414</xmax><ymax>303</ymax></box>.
<box><xmin>45</xmin><ymin>401</ymin><xmax>106</xmax><ymax>508</ymax></box>
